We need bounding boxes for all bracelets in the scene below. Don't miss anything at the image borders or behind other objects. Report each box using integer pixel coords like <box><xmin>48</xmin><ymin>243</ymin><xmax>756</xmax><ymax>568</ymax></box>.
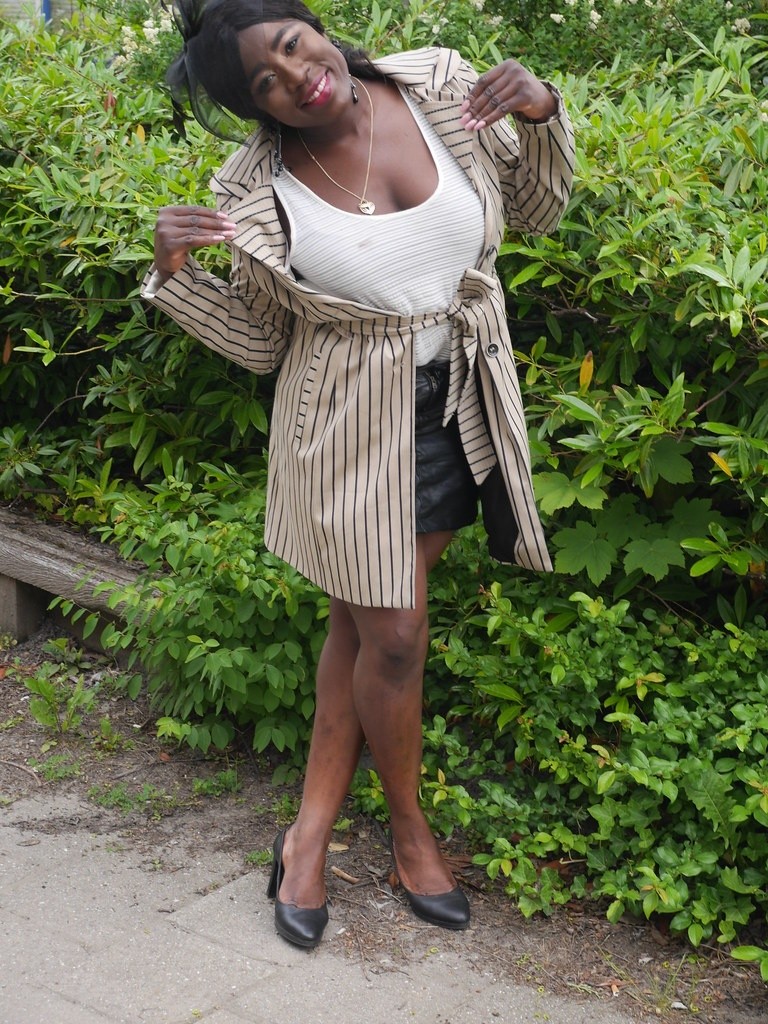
<box><xmin>297</xmin><ymin>74</ymin><xmax>376</xmax><ymax>214</ymax></box>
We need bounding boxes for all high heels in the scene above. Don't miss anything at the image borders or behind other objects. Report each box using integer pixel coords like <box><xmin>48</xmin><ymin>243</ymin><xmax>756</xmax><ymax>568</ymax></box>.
<box><xmin>266</xmin><ymin>826</ymin><xmax>328</xmax><ymax>947</ymax></box>
<box><xmin>389</xmin><ymin>828</ymin><xmax>471</xmax><ymax>929</ymax></box>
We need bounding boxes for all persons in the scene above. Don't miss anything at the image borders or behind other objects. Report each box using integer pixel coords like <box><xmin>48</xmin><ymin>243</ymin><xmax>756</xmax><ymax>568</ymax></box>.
<box><xmin>139</xmin><ymin>0</ymin><xmax>576</xmax><ymax>948</ymax></box>
